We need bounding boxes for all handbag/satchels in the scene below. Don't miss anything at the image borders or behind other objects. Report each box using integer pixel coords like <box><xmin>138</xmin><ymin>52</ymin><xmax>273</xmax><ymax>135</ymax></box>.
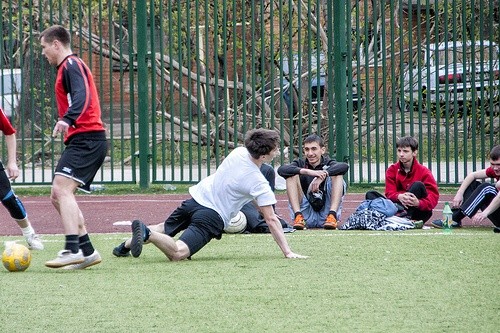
<box><xmin>340</xmin><ymin>209</ymin><xmax>386</xmax><ymax>230</ymax></box>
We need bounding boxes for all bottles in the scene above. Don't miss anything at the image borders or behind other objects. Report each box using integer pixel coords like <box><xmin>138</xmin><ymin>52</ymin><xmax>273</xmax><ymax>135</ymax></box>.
<box><xmin>442</xmin><ymin>202</ymin><xmax>453</xmax><ymax>229</ymax></box>
<box><xmin>90</xmin><ymin>185</ymin><xmax>105</xmax><ymax>191</ymax></box>
<box><xmin>163</xmin><ymin>184</ymin><xmax>176</xmax><ymax>190</ymax></box>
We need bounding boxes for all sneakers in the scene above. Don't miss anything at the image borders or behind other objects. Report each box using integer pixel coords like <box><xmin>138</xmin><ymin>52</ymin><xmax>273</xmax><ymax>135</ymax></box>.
<box><xmin>293</xmin><ymin>215</ymin><xmax>305</xmax><ymax>229</ymax></box>
<box><xmin>130</xmin><ymin>219</ymin><xmax>150</xmax><ymax>258</ymax></box>
<box><xmin>430</xmin><ymin>219</ymin><xmax>460</xmax><ymax>228</ymax></box>
<box><xmin>324</xmin><ymin>214</ymin><xmax>337</xmax><ymax>229</ymax></box>
<box><xmin>112</xmin><ymin>242</ymin><xmax>130</xmax><ymax>257</ymax></box>
<box><xmin>413</xmin><ymin>220</ymin><xmax>423</xmax><ymax>229</ymax></box>
<box><xmin>45</xmin><ymin>249</ymin><xmax>101</xmax><ymax>269</ymax></box>
<box><xmin>23</xmin><ymin>233</ymin><xmax>45</xmax><ymax>250</ymax></box>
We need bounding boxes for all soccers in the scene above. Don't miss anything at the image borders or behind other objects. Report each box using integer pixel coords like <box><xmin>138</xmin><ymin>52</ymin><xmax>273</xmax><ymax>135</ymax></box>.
<box><xmin>224</xmin><ymin>211</ymin><xmax>247</xmax><ymax>234</ymax></box>
<box><xmin>3</xmin><ymin>244</ymin><xmax>31</xmax><ymax>272</ymax></box>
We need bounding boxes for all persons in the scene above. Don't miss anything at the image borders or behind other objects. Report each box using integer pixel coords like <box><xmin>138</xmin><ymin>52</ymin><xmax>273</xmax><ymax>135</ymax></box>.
<box><xmin>277</xmin><ymin>135</ymin><xmax>349</xmax><ymax>230</ymax></box>
<box><xmin>431</xmin><ymin>145</ymin><xmax>500</xmax><ymax>228</ymax></box>
<box><xmin>365</xmin><ymin>136</ymin><xmax>439</xmax><ymax>224</ymax></box>
<box><xmin>39</xmin><ymin>25</ymin><xmax>107</xmax><ymax>269</ymax></box>
<box><xmin>112</xmin><ymin>128</ymin><xmax>313</xmax><ymax>262</ymax></box>
<box><xmin>0</xmin><ymin>107</ymin><xmax>44</xmax><ymax>250</ymax></box>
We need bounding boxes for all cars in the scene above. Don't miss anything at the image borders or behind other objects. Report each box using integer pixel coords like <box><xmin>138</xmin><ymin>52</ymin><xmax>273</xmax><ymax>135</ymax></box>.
<box><xmin>0</xmin><ymin>68</ymin><xmax>21</xmax><ymax>122</ymax></box>
<box><xmin>260</xmin><ymin>53</ymin><xmax>365</xmax><ymax>125</ymax></box>
<box><xmin>395</xmin><ymin>40</ymin><xmax>500</xmax><ymax>117</ymax></box>
